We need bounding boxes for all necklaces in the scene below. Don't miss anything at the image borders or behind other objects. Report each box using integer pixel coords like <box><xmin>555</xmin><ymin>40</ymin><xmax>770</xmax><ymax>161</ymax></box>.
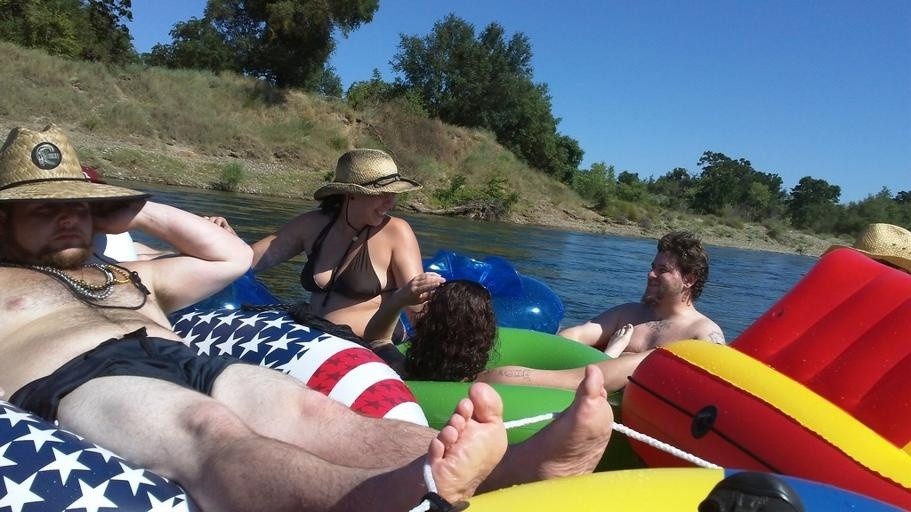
<box><xmin>26</xmin><ymin>254</ymin><xmax>150</xmax><ymax>311</ymax></box>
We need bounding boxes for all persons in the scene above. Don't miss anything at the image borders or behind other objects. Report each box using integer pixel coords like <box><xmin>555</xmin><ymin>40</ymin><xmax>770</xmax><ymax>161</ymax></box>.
<box><xmin>558</xmin><ymin>228</ymin><xmax>725</xmax><ymax>352</ymax></box>
<box><xmin>362</xmin><ymin>272</ymin><xmax>654</xmax><ymax>397</ymax></box>
<box><xmin>1</xmin><ymin>123</ymin><xmax>615</xmax><ymax>511</ymax></box>
<box><xmin>201</xmin><ymin>149</ymin><xmax>428</xmax><ymax>340</ymax></box>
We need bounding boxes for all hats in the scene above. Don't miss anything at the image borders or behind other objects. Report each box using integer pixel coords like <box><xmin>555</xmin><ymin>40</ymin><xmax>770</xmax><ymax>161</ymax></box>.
<box><xmin>0</xmin><ymin>124</ymin><xmax>150</xmax><ymax>201</ymax></box>
<box><xmin>314</xmin><ymin>148</ymin><xmax>423</xmax><ymax>200</ymax></box>
